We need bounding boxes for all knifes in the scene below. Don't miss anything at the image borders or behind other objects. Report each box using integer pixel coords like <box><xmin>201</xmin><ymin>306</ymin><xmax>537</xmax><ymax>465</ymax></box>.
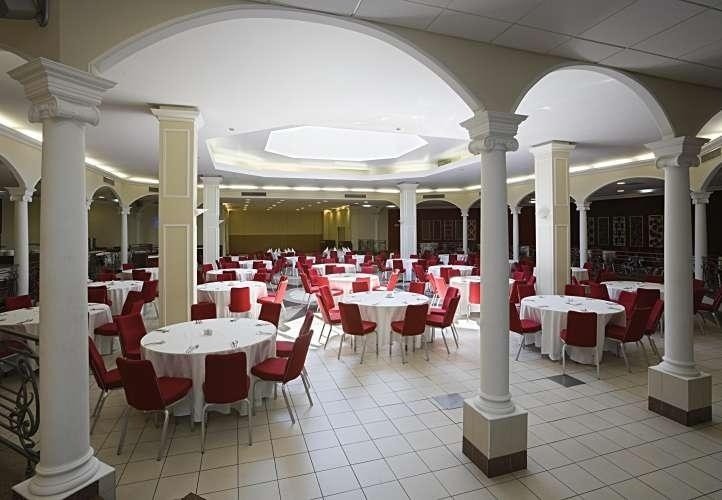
<box><xmin>187</xmin><ymin>344</ymin><xmax>200</xmax><ymax>358</ymax></box>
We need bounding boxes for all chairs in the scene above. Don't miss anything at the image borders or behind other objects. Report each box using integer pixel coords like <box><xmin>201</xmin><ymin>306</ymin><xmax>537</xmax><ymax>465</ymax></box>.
<box><xmin>390</xmin><ymin>303</ymin><xmax>429</xmax><ymax>364</ymax></box>
<box><xmin>121</xmin><ymin>291</ymin><xmax>146</xmax><ymax>314</ymax></box>
<box><xmin>223</xmin><ymin>271</ymin><xmax>236</xmax><ymax>281</ymax></box>
<box><xmin>200</xmin><ymin>352</ymin><xmax>252</xmax><ymax>453</ymax></box>
<box><xmin>448</xmin><ymin>269</ymin><xmax>460</xmax><ymax>278</ymax></box>
<box><xmin>115</xmin><ymin>313</ymin><xmax>147</xmax><ymax>360</ymax></box>
<box><xmin>361</xmin><ymin>267</ymin><xmax>373</xmax><ymax>273</ymax></box>
<box><xmin>566</xmin><ymin>285</ymin><xmax>585</xmax><ymax>297</ymax></box>
<box><xmin>409</xmin><ymin>282</ymin><xmax>426</xmax><ymax>294</ymax></box>
<box><xmin>440</xmin><ymin>268</ymin><xmax>452</xmax><ymax>284</ymax></box>
<box><xmin>603</xmin><ymin>292</ymin><xmax>656</xmax><ymax>372</ymax></box>
<box><xmin>560</xmin><ymin>310</ymin><xmax>600</xmax><ymax>380</ymax></box>
<box><xmin>351</xmin><ymin>282</ymin><xmax>369</xmax><ymax>294</ymax></box>
<box><xmin>228</xmin><ymin>287</ymin><xmax>251</xmax><ymax>317</ymax></box>
<box><xmin>448</xmin><ymin>254</ymin><xmax>457</xmax><ymax>265</ymax></box>
<box><xmin>268</xmin><ymin>276</ymin><xmax>288</xmax><ymax>311</ymax></box>
<box><xmin>415</xmin><ymin>265</ymin><xmax>428</xmax><ymax>282</ymax></box>
<box><xmin>202</xmin><ymin>264</ymin><xmax>213</xmax><ymax>279</ymax></box>
<box><xmin>132</xmin><ymin>269</ymin><xmax>145</xmax><ymax>281</ymax></box>
<box><xmin>517</xmin><ymin>284</ymin><xmax>534</xmax><ymax>301</ymax></box>
<box><xmin>259</xmin><ymin>301</ymin><xmax>281</xmax><ymax>328</ymax></box>
<box><xmin>469</xmin><ymin>282</ymin><xmax>480</xmax><ymax>305</ymax></box>
<box><xmin>326</xmin><ymin>265</ymin><xmax>336</xmax><ymax>274</ymax></box>
<box><xmin>316</xmin><ymin>294</ymin><xmax>345</xmax><ymax>348</ymax></box>
<box><xmin>276</xmin><ymin>309</ymin><xmax>314</xmax><ymax>387</ymax></box>
<box><xmin>510</xmin><ymin>301</ymin><xmax>542</xmax><ymax>361</ymax></box>
<box><xmin>332</xmin><ymin>267</ymin><xmax>345</xmax><ymax>273</ymax></box>
<box><xmin>319</xmin><ymin>285</ymin><xmax>339</xmax><ymax>314</ymax></box>
<box><xmin>253</xmin><ymin>262</ymin><xmax>263</xmax><ymax>269</ymax></box>
<box><xmin>694</xmin><ymin>287</ymin><xmax>709</xmax><ymax>336</ymax></box>
<box><xmin>253</xmin><ymin>329</ymin><xmax>314</xmax><ymax>424</ymax></box>
<box><xmin>431</xmin><ymin>288</ymin><xmax>459</xmax><ymax>338</ymax></box>
<box><xmin>141</xmin><ymin>280</ymin><xmax>159</xmax><ymax>318</ymax></box>
<box><xmin>257</xmin><ymin>280</ymin><xmax>288</xmax><ymax>304</ymax></box>
<box><xmin>88</xmin><ymin>285</ymin><xmax>112</xmax><ymax>306</ymax></box>
<box><xmin>699</xmin><ymin>290</ymin><xmax>721</xmax><ymax>329</ymax></box>
<box><xmin>338</xmin><ymin>302</ymin><xmax>379</xmax><ymax>363</ymax></box>
<box><xmin>393</xmin><ymin>260</ymin><xmax>406</xmax><ymax>287</ymax></box>
<box><xmin>116</xmin><ymin>357</ymin><xmax>194</xmax><ymax>461</ymax></box>
<box><xmin>426</xmin><ymin>295</ymin><xmax>460</xmax><ymax>354</ymax></box>
<box><xmin>5</xmin><ymin>295</ymin><xmax>32</xmax><ymax>311</ymax></box>
<box><xmin>296</xmin><ymin>262</ymin><xmax>303</xmax><ymax>272</ymax></box>
<box><xmin>299</xmin><ymin>272</ymin><xmax>320</xmax><ymax>310</ymax></box>
<box><xmin>372</xmin><ymin>268</ymin><xmax>400</xmax><ymax>292</ymax></box>
<box><xmin>645</xmin><ymin>300</ymin><xmax>664</xmax><ymax>362</ymax></box>
<box><xmin>89</xmin><ymin>336</ymin><xmax>125</xmax><ymax>437</ymax></box>
<box><xmin>589</xmin><ymin>282</ymin><xmax>610</xmax><ymax>299</ymax></box>
<box><xmin>583</xmin><ymin>262</ymin><xmax>593</xmax><ymax>279</ymax></box>
<box><xmin>514</xmin><ymin>271</ymin><xmax>526</xmax><ymax>280</ymax></box>
<box><xmin>191</xmin><ymin>304</ymin><xmax>216</xmax><ymax>321</ymax></box>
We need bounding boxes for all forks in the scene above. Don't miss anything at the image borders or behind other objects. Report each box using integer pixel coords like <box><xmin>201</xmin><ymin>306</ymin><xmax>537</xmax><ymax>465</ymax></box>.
<box><xmin>185</xmin><ymin>344</ymin><xmax>191</xmax><ymax>351</ymax></box>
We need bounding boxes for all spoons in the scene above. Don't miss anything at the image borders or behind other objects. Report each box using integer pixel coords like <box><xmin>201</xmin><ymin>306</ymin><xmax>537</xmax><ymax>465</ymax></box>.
<box><xmin>233</xmin><ymin>338</ymin><xmax>241</xmax><ymax>351</ymax></box>
